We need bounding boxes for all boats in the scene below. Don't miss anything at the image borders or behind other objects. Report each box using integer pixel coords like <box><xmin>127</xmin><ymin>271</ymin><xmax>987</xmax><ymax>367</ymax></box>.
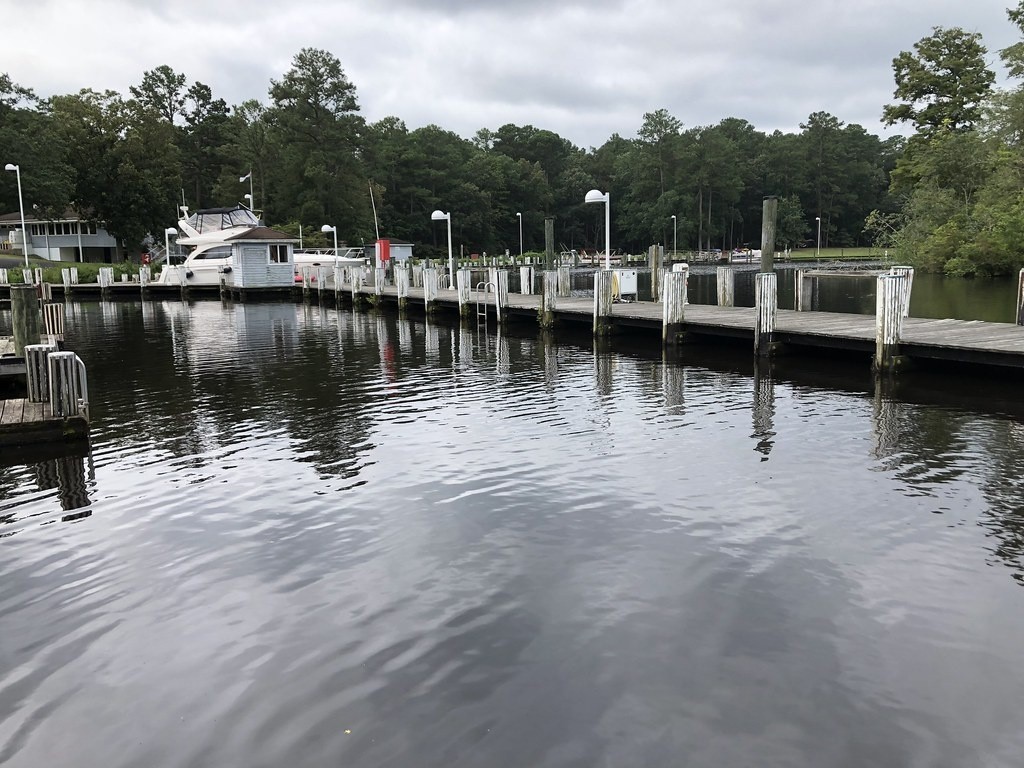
<box><xmin>727</xmin><ymin>249</ymin><xmax>762</xmax><ymax>264</ymax></box>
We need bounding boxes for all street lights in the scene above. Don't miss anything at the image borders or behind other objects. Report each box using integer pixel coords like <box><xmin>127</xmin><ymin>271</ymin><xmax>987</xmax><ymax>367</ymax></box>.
<box><xmin>816</xmin><ymin>217</ymin><xmax>821</xmax><ymax>261</ymax></box>
<box><xmin>321</xmin><ymin>225</ymin><xmax>341</xmax><ymax>287</ymax></box>
<box><xmin>431</xmin><ymin>210</ymin><xmax>456</xmax><ymax>291</ymax></box>
<box><xmin>516</xmin><ymin>212</ymin><xmax>524</xmax><ymax>257</ymax></box>
<box><xmin>584</xmin><ymin>189</ymin><xmax>612</xmax><ymax>269</ymax></box>
<box><xmin>165</xmin><ymin>228</ymin><xmax>178</xmax><ymax>266</ymax></box>
<box><xmin>245</xmin><ymin>194</ymin><xmax>254</xmax><ymax>209</ymax></box>
<box><xmin>5</xmin><ymin>164</ymin><xmax>28</xmax><ymax>268</ymax></box>
<box><xmin>671</xmin><ymin>215</ymin><xmax>676</xmax><ymax>258</ymax></box>
